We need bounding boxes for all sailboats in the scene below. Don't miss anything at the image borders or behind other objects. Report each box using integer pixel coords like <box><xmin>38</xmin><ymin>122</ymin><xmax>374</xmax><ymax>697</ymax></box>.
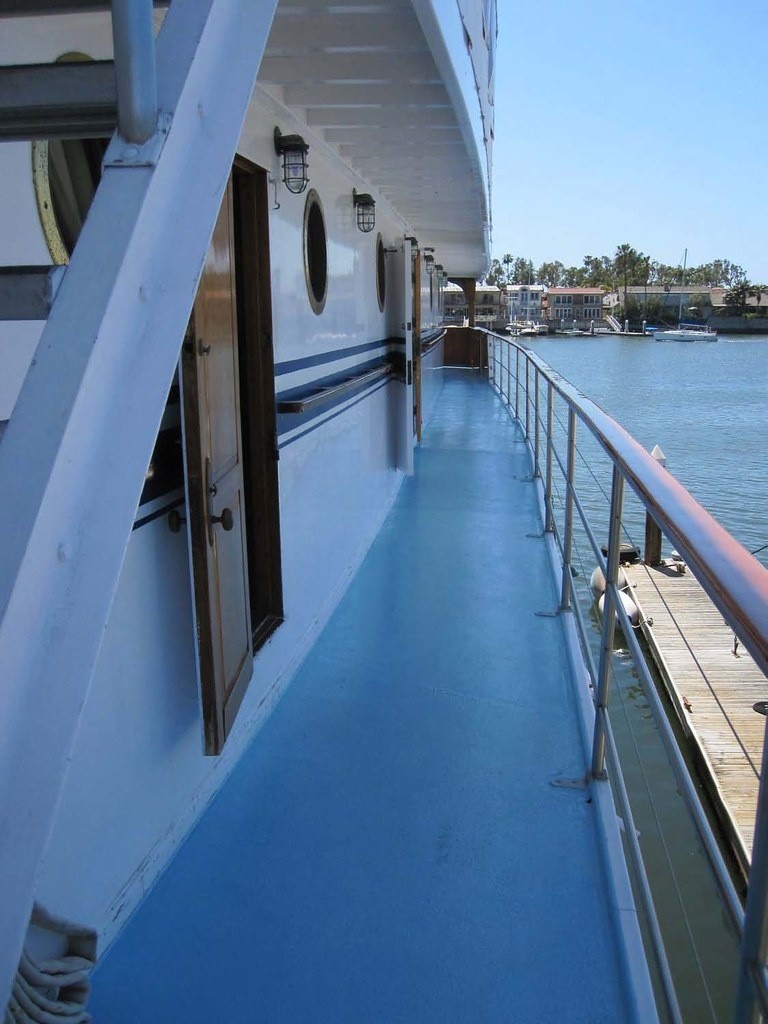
<box><xmin>650</xmin><ymin>249</ymin><xmax>717</xmax><ymax>341</ymax></box>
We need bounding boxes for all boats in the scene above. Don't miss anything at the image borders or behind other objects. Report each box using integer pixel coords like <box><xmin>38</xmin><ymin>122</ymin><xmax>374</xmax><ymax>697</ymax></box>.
<box><xmin>0</xmin><ymin>0</ymin><xmax>768</xmax><ymax>1024</ymax></box>
<box><xmin>504</xmin><ymin>322</ymin><xmax>548</xmax><ymax>337</ymax></box>
